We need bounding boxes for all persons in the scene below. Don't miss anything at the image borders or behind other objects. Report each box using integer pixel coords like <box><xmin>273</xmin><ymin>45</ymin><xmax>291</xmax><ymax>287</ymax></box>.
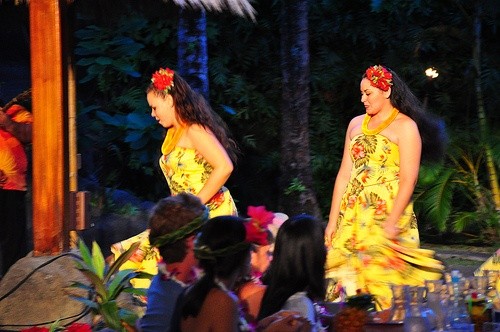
<box><xmin>111</xmin><ymin>68</ymin><xmax>327</xmax><ymax>332</ymax></box>
<box><xmin>0</xmin><ymin>103</ymin><xmax>33</xmax><ymax>279</ymax></box>
<box><xmin>324</xmin><ymin>65</ymin><xmax>447</xmax><ymax>312</ymax></box>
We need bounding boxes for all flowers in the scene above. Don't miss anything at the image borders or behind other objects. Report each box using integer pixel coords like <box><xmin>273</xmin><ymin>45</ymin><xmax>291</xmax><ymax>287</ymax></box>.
<box><xmin>245</xmin><ymin>205</ymin><xmax>276</xmax><ymax>247</ymax></box>
<box><xmin>151</xmin><ymin>68</ymin><xmax>176</xmax><ymax>95</ymax></box>
<box><xmin>366</xmin><ymin>65</ymin><xmax>393</xmax><ymax>92</ymax></box>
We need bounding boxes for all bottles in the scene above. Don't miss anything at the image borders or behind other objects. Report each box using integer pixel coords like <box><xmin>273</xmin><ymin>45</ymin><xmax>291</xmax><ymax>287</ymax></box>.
<box><xmin>392</xmin><ymin>270</ymin><xmax>500</xmax><ymax>332</ymax></box>
